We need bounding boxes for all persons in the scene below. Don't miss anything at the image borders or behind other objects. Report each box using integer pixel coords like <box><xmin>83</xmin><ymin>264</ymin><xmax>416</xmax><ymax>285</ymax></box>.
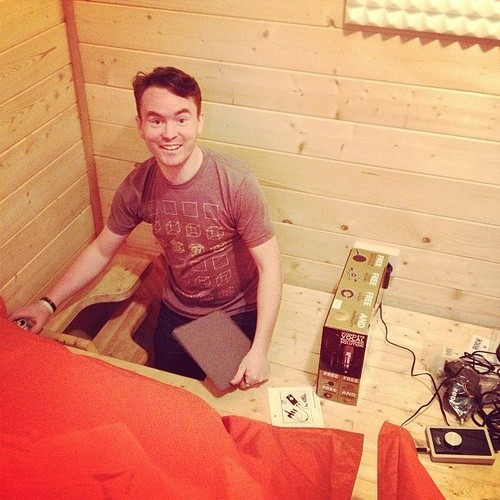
<box><xmin>9</xmin><ymin>66</ymin><xmax>284</xmax><ymax>392</ymax></box>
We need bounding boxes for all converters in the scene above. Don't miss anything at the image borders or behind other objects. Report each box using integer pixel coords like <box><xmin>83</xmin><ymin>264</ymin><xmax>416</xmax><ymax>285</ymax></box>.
<box><xmin>423</xmin><ymin>426</ymin><xmax>496</xmax><ymax>465</ymax></box>
<box><xmin>445</xmin><ymin>361</ymin><xmax>482</xmax><ymax>418</ymax></box>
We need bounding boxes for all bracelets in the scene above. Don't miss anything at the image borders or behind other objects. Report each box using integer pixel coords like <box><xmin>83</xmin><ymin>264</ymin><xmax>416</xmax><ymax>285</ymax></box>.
<box><xmin>38</xmin><ymin>296</ymin><xmax>58</xmax><ymax>315</ymax></box>
